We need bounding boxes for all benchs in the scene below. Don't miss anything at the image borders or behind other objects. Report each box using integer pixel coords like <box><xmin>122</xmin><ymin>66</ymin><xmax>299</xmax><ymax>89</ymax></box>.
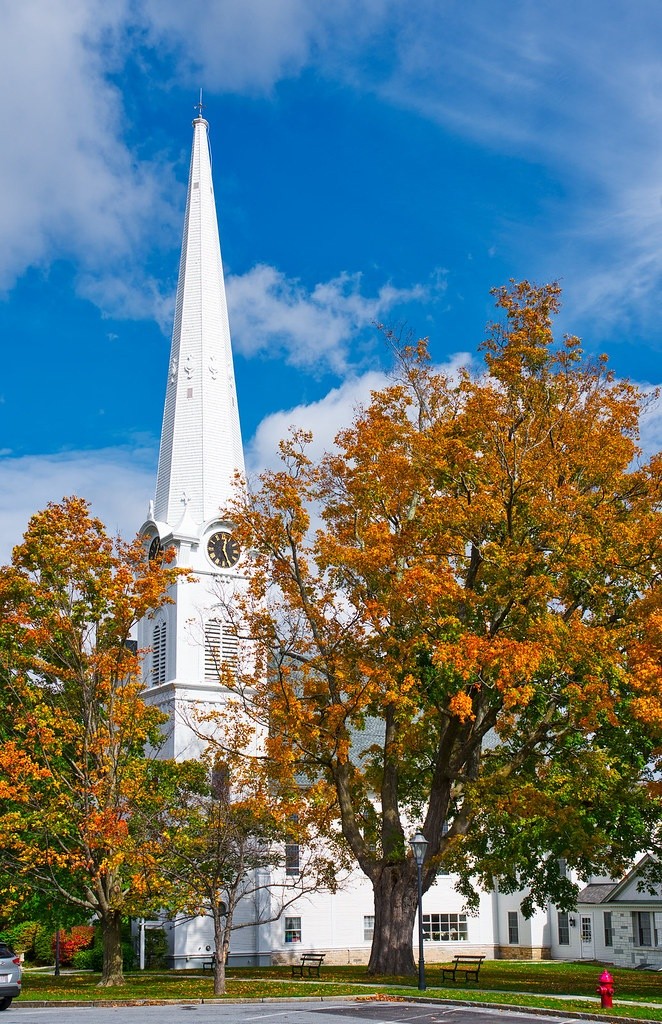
<box><xmin>440</xmin><ymin>954</ymin><xmax>486</xmax><ymax>984</ymax></box>
<box><xmin>290</xmin><ymin>953</ymin><xmax>326</xmax><ymax>978</ymax></box>
<box><xmin>203</xmin><ymin>952</ymin><xmax>231</xmax><ymax>972</ymax></box>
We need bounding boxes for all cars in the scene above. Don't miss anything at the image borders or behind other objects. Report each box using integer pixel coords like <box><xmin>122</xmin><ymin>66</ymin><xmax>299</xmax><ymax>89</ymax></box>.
<box><xmin>0</xmin><ymin>941</ymin><xmax>23</xmax><ymax>1010</ymax></box>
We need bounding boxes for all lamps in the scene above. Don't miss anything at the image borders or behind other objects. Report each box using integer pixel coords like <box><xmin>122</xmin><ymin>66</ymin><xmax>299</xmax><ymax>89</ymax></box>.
<box><xmin>569</xmin><ymin>917</ymin><xmax>576</xmax><ymax>928</ymax></box>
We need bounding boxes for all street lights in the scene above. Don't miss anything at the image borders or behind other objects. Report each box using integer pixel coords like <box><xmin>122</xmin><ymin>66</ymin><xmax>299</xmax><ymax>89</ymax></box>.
<box><xmin>406</xmin><ymin>829</ymin><xmax>431</xmax><ymax>991</ymax></box>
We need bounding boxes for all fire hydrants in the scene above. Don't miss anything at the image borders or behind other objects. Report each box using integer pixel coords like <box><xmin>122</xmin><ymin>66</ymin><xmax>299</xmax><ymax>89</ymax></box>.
<box><xmin>595</xmin><ymin>969</ymin><xmax>615</xmax><ymax>1009</ymax></box>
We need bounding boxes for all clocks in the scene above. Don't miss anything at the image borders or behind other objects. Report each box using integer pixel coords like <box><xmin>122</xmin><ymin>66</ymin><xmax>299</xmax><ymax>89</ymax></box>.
<box><xmin>148</xmin><ymin>536</ymin><xmax>165</xmax><ymax>572</ymax></box>
<box><xmin>207</xmin><ymin>531</ymin><xmax>240</xmax><ymax>569</ymax></box>
